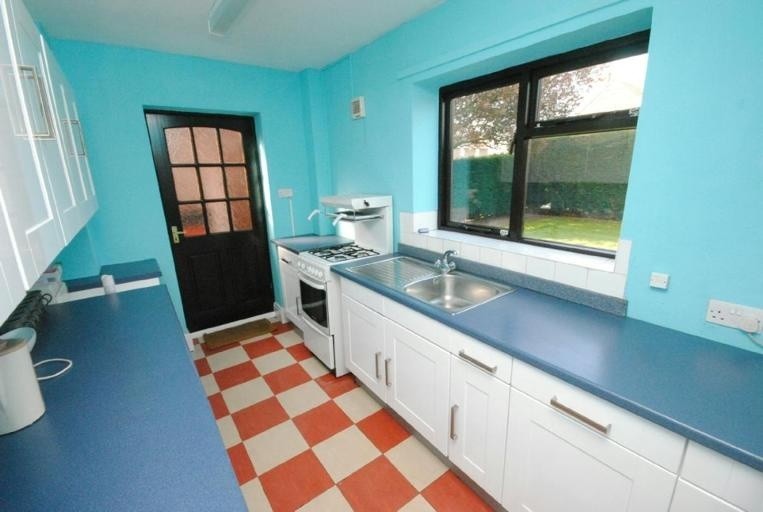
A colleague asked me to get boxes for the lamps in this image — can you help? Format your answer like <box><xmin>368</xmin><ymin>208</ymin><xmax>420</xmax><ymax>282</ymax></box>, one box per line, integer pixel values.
<box><xmin>207</xmin><ymin>2</ymin><xmax>249</xmax><ymax>37</ymax></box>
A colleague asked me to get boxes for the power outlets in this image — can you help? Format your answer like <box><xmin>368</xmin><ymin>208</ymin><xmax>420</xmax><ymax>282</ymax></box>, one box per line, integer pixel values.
<box><xmin>706</xmin><ymin>299</ymin><xmax>763</xmax><ymax>334</ymax></box>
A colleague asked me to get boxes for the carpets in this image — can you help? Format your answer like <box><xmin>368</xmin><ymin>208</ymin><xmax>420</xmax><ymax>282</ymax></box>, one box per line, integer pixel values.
<box><xmin>204</xmin><ymin>318</ymin><xmax>277</xmax><ymax>350</ymax></box>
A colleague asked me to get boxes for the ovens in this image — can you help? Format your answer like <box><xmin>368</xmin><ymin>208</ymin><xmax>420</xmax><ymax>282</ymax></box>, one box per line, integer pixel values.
<box><xmin>296</xmin><ymin>259</ymin><xmax>332</xmax><ymax>335</ymax></box>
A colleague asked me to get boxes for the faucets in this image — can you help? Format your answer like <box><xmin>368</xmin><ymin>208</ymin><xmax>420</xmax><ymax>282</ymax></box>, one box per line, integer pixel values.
<box><xmin>435</xmin><ymin>251</ymin><xmax>457</xmax><ymax>274</ymax></box>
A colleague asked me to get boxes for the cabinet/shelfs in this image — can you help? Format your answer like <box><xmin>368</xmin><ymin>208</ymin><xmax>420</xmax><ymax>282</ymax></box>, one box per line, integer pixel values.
<box><xmin>447</xmin><ymin>325</ymin><xmax>514</xmax><ymax>512</ymax></box>
<box><xmin>668</xmin><ymin>439</ymin><xmax>763</xmax><ymax>512</ymax></box>
<box><xmin>1</xmin><ymin>0</ymin><xmax>99</xmax><ymax>329</ymax></box>
<box><xmin>500</xmin><ymin>357</ymin><xmax>688</xmax><ymax>512</ymax></box>
<box><xmin>339</xmin><ymin>277</ymin><xmax>447</xmax><ymax>468</ymax></box>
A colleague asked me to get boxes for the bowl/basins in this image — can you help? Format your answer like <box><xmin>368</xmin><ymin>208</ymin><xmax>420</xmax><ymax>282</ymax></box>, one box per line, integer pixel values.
<box><xmin>0</xmin><ymin>327</ymin><xmax>37</xmax><ymax>354</ymax></box>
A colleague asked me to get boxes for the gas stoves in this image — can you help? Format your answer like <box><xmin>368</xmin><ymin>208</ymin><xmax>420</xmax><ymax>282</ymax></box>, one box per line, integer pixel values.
<box><xmin>299</xmin><ymin>242</ymin><xmax>387</xmax><ymax>267</ymax></box>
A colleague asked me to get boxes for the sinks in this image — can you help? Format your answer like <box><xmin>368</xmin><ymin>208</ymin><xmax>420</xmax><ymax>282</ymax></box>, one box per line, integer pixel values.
<box><xmin>403</xmin><ymin>275</ymin><xmax>517</xmax><ymax>316</ymax></box>
